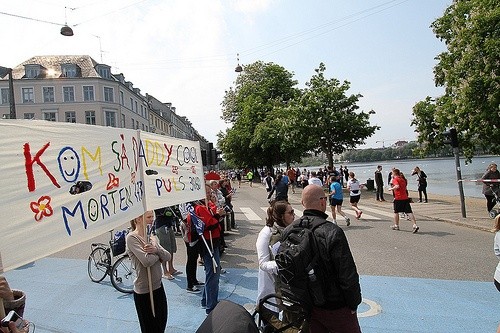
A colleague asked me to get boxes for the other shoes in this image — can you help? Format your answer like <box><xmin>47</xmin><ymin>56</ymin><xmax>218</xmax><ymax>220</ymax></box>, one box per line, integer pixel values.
<box><xmin>201</xmin><ymin>304</ymin><xmax>213</xmax><ymax>315</ymax></box>
<box><xmin>390</xmin><ymin>225</ymin><xmax>399</xmax><ymax>230</ymax></box>
<box><xmin>220</xmin><ymin>269</ymin><xmax>226</xmax><ymax>274</ymax></box>
<box><xmin>345</xmin><ymin>218</ymin><xmax>350</xmax><ymax>226</ymax></box>
<box><xmin>411</xmin><ymin>225</ymin><xmax>419</xmax><ymax>234</ymax></box>
<box><xmin>356</xmin><ymin>211</ymin><xmax>362</xmax><ymax>218</ymax></box>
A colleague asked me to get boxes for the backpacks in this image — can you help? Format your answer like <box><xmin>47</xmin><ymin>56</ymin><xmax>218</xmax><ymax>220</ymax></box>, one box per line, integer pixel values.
<box><xmin>274</xmin><ymin>216</ymin><xmax>332</xmax><ymax>312</ymax></box>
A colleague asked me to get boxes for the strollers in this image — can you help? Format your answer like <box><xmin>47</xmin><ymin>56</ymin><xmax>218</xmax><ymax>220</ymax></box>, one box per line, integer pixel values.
<box><xmin>488</xmin><ymin>184</ymin><xmax>500</xmax><ymax>219</ymax></box>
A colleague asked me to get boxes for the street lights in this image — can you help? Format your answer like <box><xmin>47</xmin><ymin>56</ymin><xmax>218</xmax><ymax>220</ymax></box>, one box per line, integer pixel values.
<box><xmin>0</xmin><ymin>65</ymin><xmax>17</xmax><ymax>120</ymax></box>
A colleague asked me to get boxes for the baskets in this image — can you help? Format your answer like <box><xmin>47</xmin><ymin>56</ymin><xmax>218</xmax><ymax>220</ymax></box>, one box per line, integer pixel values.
<box><xmin>110</xmin><ymin>239</ymin><xmax>126</xmax><ymax>257</ymax></box>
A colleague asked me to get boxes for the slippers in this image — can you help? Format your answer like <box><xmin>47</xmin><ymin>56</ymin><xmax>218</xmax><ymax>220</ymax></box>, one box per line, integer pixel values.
<box><xmin>163</xmin><ymin>274</ymin><xmax>175</xmax><ymax>280</ymax></box>
<box><xmin>172</xmin><ymin>270</ymin><xmax>183</xmax><ymax>274</ymax></box>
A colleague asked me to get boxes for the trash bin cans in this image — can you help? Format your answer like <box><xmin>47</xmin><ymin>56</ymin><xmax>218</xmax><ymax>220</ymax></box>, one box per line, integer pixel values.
<box><xmin>367</xmin><ymin>180</ymin><xmax>374</xmax><ymax>191</ymax></box>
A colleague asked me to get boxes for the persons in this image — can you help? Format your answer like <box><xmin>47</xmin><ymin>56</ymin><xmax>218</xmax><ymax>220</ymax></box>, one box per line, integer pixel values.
<box><xmin>126</xmin><ymin>164</ymin><xmax>411</xmax><ymax>333</ymax></box>
<box><xmin>493</xmin><ymin>214</ymin><xmax>500</xmax><ymax>293</ymax></box>
<box><xmin>0</xmin><ymin>277</ymin><xmax>32</xmax><ymax>333</ymax></box>
<box><xmin>411</xmin><ymin>166</ymin><xmax>428</xmax><ymax>203</ymax></box>
<box><xmin>374</xmin><ymin>165</ymin><xmax>386</xmax><ymax>202</ymax></box>
<box><xmin>276</xmin><ymin>185</ymin><xmax>362</xmax><ymax>333</ymax></box>
<box><xmin>328</xmin><ymin>176</ymin><xmax>350</xmax><ymax>226</ymax></box>
<box><xmin>481</xmin><ymin>162</ymin><xmax>500</xmax><ymax>220</ymax></box>
<box><xmin>389</xmin><ymin>169</ymin><xmax>419</xmax><ymax>234</ymax></box>
<box><xmin>256</xmin><ymin>200</ymin><xmax>296</xmax><ymax>333</ymax></box>
<box><xmin>346</xmin><ymin>172</ymin><xmax>362</xmax><ymax>220</ymax></box>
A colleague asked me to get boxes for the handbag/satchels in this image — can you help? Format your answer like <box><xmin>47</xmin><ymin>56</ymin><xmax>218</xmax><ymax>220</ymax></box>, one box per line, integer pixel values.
<box><xmin>352</xmin><ymin>190</ymin><xmax>359</xmax><ymax>194</ymax></box>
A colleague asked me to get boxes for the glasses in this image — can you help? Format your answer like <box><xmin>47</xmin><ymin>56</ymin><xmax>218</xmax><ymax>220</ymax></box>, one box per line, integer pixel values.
<box><xmin>283</xmin><ymin>209</ymin><xmax>294</xmax><ymax>216</ymax></box>
<box><xmin>320</xmin><ymin>197</ymin><xmax>327</xmax><ymax>201</ymax></box>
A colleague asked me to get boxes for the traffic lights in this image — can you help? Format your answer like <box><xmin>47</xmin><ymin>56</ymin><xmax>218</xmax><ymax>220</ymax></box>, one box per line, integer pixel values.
<box><xmin>441</xmin><ymin>128</ymin><xmax>455</xmax><ymax>148</ymax></box>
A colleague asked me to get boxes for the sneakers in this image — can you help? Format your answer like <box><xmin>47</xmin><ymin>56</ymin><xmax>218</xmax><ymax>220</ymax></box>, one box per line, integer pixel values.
<box><xmin>186</xmin><ymin>285</ymin><xmax>202</xmax><ymax>294</ymax></box>
<box><xmin>195</xmin><ymin>281</ymin><xmax>206</xmax><ymax>286</ymax></box>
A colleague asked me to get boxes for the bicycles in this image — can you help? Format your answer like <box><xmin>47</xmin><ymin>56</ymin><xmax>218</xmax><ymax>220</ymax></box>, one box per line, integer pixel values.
<box><xmin>86</xmin><ymin>230</ymin><xmax>137</xmax><ymax>294</ymax></box>
